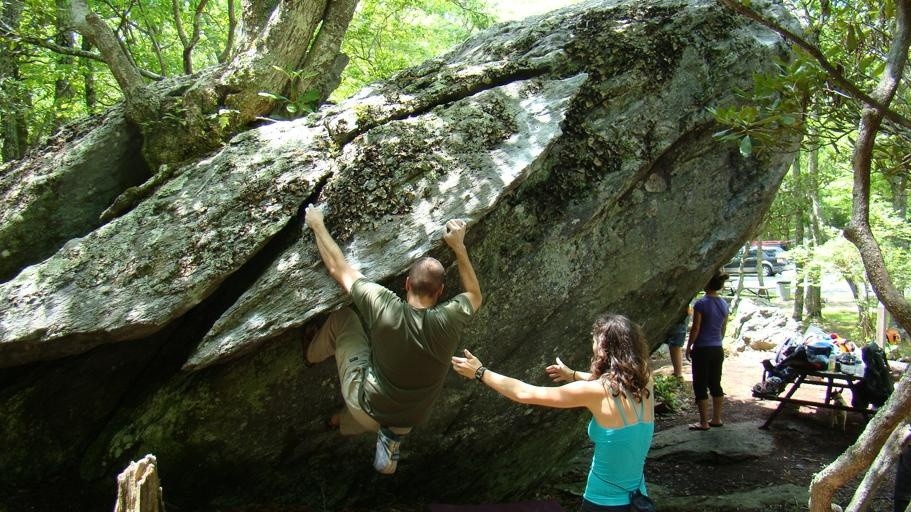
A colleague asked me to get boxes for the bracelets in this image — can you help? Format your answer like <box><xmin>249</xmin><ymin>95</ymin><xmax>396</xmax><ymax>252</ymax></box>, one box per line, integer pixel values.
<box><xmin>572</xmin><ymin>371</ymin><xmax>576</xmax><ymax>382</ymax></box>
<box><xmin>475</xmin><ymin>365</ymin><xmax>487</xmax><ymax>383</ymax></box>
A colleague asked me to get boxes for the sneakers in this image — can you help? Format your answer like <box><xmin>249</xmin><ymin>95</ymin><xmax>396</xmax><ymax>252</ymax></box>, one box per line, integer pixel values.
<box><xmin>303</xmin><ymin>323</ymin><xmax>318</xmax><ymax>368</ymax></box>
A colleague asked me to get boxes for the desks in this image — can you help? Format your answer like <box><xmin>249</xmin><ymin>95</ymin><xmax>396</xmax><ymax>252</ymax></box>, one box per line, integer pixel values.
<box><xmin>759</xmin><ymin>364</ymin><xmax>877</xmax><ymax>430</ymax></box>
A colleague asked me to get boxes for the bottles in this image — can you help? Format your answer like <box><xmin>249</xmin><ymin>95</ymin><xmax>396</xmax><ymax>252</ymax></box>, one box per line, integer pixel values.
<box><xmin>855</xmin><ymin>360</ymin><xmax>862</xmax><ymax>377</ymax></box>
<box><xmin>826</xmin><ymin>351</ymin><xmax>836</xmax><ymax>372</ymax></box>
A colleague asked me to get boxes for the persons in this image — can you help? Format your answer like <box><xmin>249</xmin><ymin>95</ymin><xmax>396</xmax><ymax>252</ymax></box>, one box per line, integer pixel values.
<box><xmin>302</xmin><ymin>202</ymin><xmax>483</xmax><ymax>476</ymax></box>
<box><xmin>684</xmin><ymin>270</ymin><xmax>730</xmax><ymax>430</ymax></box>
<box><xmin>665</xmin><ymin>303</ymin><xmax>694</xmax><ymax>379</ymax></box>
<box><xmin>450</xmin><ymin>313</ymin><xmax>658</xmax><ymax>512</ymax></box>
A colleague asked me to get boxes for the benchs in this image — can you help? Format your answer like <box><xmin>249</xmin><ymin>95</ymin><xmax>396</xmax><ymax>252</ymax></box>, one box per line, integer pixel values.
<box><xmin>717</xmin><ymin>279</ymin><xmax>779</xmax><ymax>307</ymax></box>
<box><xmin>752</xmin><ymin>382</ymin><xmax>789</xmax><ymax>401</ymax></box>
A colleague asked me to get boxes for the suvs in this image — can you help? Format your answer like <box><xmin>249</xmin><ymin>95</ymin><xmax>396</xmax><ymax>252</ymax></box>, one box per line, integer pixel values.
<box><xmin>722</xmin><ymin>246</ymin><xmax>794</xmax><ymax>275</ymax></box>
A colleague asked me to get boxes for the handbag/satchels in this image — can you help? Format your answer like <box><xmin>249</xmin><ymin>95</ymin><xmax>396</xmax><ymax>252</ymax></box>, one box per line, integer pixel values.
<box><xmin>373</xmin><ymin>424</ymin><xmax>401</xmax><ymax>474</ymax></box>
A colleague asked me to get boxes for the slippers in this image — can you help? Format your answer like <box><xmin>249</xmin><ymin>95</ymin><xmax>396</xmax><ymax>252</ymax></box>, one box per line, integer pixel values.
<box><xmin>688</xmin><ymin>420</ymin><xmax>722</xmax><ymax>431</ymax></box>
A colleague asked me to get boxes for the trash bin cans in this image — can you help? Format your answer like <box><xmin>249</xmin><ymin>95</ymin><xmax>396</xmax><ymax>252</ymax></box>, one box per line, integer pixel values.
<box><xmin>776</xmin><ymin>281</ymin><xmax>791</xmax><ymax>301</ymax></box>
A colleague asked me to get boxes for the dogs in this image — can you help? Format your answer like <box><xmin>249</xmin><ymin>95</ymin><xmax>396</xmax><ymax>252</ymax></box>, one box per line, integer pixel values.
<box><xmin>829</xmin><ymin>386</ymin><xmax>848</xmax><ymax>432</ymax></box>
